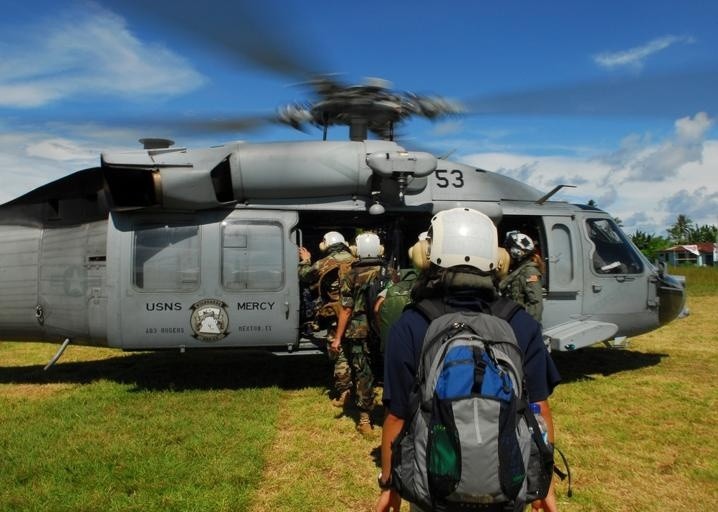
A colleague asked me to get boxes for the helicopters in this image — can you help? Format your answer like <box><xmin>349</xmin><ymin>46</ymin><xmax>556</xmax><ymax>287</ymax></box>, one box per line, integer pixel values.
<box><xmin>0</xmin><ymin>0</ymin><xmax>690</xmax><ymax>376</ymax></box>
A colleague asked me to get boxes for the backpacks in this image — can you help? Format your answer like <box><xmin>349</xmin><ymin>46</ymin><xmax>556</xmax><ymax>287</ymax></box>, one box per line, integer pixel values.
<box><xmin>364</xmin><ymin>268</ymin><xmax>391</xmax><ymax>335</ymax></box>
<box><xmin>318</xmin><ymin>258</ymin><xmax>347</xmax><ymax>318</ymax></box>
<box><xmin>380</xmin><ymin>271</ymin><xmax>422</xmax><ymax>352</ymax></box>
<box><xmin>393</xmin><ymin>298</ymin><xmax>554</xmax><ymax>511</ymax></box>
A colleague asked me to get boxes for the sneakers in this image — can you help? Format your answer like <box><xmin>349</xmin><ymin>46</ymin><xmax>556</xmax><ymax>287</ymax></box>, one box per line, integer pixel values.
<box><xmin>333</xmin><ymin>388</ymin><xmax>375</xmax><ymax>442</ymax></box>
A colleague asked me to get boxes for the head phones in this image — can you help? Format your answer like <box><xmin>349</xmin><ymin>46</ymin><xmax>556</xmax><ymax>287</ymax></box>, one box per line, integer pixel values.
<box><xmin>510</xmin><ymin>247</ymin><xmax>524</xmax><ymax>260</ymax></box>
<box><xmin>319</xmin><ymin>240</ymin><xmax>349</xmax><ymax>253</ymax></box>
<box><xmin>407</xmin><ymin>225</ymin><xmax>511</xmax><ymax>277</ymax></box>
<box><xmin>349</xmin><ymin>244</ymin><xmax>385</xmax><ymax>258</ymax></box>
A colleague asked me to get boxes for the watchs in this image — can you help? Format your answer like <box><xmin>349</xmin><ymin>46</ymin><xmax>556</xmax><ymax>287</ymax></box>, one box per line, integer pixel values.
<box><xmin>376</xmin><ymin>473</ymin><xmax>390</xmax><ymax>491</ymax></box>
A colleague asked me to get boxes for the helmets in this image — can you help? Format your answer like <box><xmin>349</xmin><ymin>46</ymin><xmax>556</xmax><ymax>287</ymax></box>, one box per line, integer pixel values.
<box><xmin>323</xmin><ymin>231</ymin><xmax>346</xmax><ymax>247</ymax></box>
<box><xmin>426</xmin><ymin>207</ymin><xmax>501</xmax><ymax>273</ymax></box>
<box><xmin>355</xmin><ymin>232</ymin><xmax>381</xmax><ymax>259</ymax></box>
<box><xmin>507</xmin><ymin>232</ymin><xmax>535</xmax><ymax>264</ymax></box>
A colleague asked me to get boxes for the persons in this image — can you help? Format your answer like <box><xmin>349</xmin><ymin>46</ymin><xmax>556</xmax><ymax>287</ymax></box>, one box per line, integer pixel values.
<box><xmin>372</xmin><ymin>238</ymin><xmax>429</xmax><ymax>359</ymax></box>
<box><xmin>375</xmin><ymin>206</ymin><xmax>566</xmax><ymax>512</ymax></box>
<box><xmin>297</xmin><ymin>229</ymin><xmax>355</xmax><ymax>409</ymax></box>
<box><xmin>299</xmin><ymin>283</ymin><xmax>313</xmax><ymax>340</ymax></box>
<box><xmin>329</xmin><ymin>232</ymin><xmax>395</xmax><ymax>439</ymax></box>
<box><xmin>498</xmin><ymin>230</ymin><xmax>546</xmax><ymax>324</ymax></box>
<box><xmin>375</xmin><ymin>268</ymin><xmax>415</xmax><ymax>313</ymax></box>
